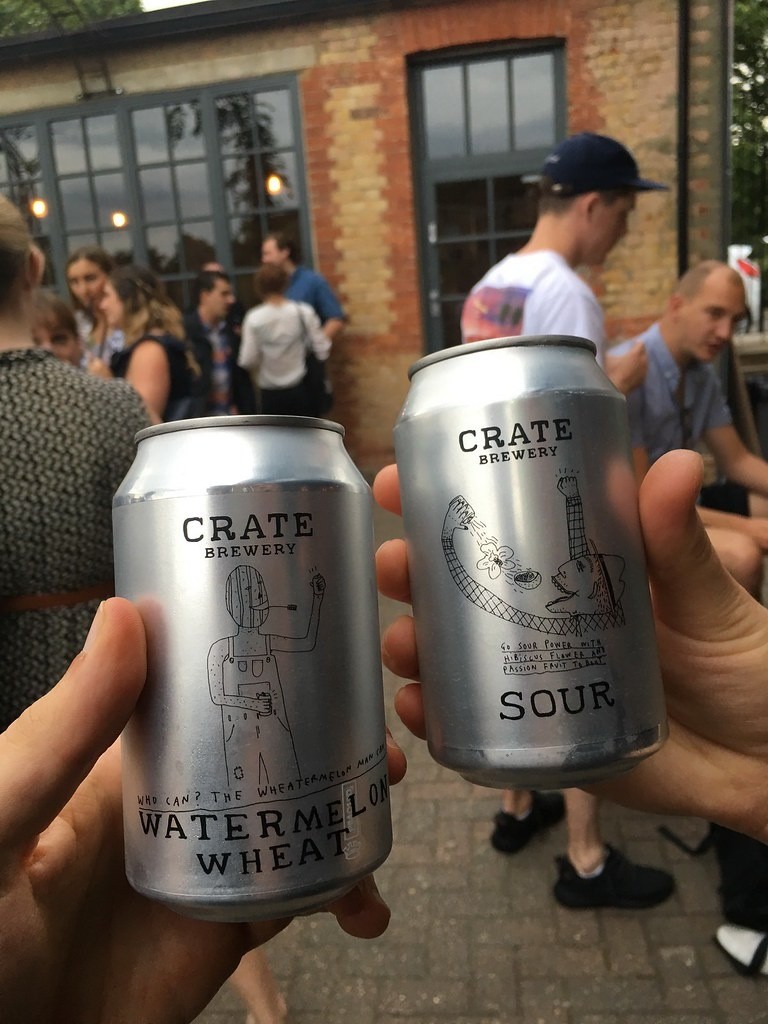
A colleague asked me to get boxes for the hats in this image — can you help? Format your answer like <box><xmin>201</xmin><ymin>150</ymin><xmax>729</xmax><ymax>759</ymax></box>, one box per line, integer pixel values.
<box><xmin>537</xmin><ymin>130</ymin><xmax>670</xmax><ymax>193</ymax></box>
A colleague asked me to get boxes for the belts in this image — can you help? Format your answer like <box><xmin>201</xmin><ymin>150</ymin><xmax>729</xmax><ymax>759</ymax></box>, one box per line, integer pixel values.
<box><xmin>0</xmin><ymin>581</ymin><xmax>115</xmax><ymax>612</ymax></box>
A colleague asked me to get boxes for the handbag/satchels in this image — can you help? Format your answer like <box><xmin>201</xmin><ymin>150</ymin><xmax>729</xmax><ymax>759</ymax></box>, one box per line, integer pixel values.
<box><xmin>302</xmin><ymin>341</ymin><xmax>335</xmax><ymax>418</ymax></box>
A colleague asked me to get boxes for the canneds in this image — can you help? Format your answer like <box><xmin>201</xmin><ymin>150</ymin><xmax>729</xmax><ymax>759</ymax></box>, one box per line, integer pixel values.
<box><xmin>390</xmin><ymin>334</ymin><xmax>668</xmax><ymax>792</ymax></box>
<box><xmin>111</xmin><ymin>414</ymin><xmax>392</xmax><ymax>924</ymax></box>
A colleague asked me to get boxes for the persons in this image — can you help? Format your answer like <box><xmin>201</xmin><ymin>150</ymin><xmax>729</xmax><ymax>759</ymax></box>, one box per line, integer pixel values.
<box><xmin>31</xmin><ymin>234</ymin><xmax>349</xmax><ymax>427</ymax></box>
<box><xmin>0</xmin><ymin>195</ymin><xmax>152</xmax><ymax>733</ymax></box>
<box><xmin>458</xmin><ymin>133</ymin><xmax>675</xmax><ymax>911</ymax></box>
<box><xmin>604</xmin><ymin>261</ymin><xmax>766</xmax><ymax>607</ymax></box>
<box><xmin>0</xmin><ymin>448</ymin><xmax>767</xmax><ymax>1024</ymax></box>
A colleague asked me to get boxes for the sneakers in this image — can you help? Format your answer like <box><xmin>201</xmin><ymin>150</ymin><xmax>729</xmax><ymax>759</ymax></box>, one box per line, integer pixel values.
<box><xmin>552</xmin><ymin>842</ymin><xmax>677</xmax><ymax>912</ymax></box>
<box><xmin>489</xmin><ymin>790</ymin><xmax>568</xmax><ymax>858</ymax></box>
<box><xmin>712</xmin><ymin>921</ymin><xmax>767</xmax><ymax>980</ymax></box>
